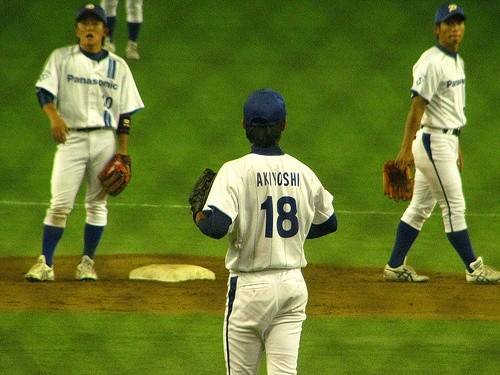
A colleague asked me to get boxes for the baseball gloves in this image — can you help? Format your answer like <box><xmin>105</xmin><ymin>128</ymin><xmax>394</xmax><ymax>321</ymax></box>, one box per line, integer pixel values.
<box><xmin>188</xmin><ymin>168</ymin><xmax>218</xmax><ymax>213</ymax></box>
<box><xmin>382</xmin><ymin>157</ymin><xmax>414</xmax><ymax>202</ymax></box>
<box><xmin>97</xmin><ymin>153</ymin><xmax>132</xmax><ymax>197</ymax></box>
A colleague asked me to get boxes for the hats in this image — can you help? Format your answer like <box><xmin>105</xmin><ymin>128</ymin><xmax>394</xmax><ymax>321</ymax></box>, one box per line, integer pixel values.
<box><xmin>76</xmin><ymin>4</ymin><xmax>106</xmax><ymax>23</ymax></box>
<box><xmin>243</xmin><ymin>89</ymin><xmax>286</xmax><ymax>128</ymax></box>
<box><xmin>434</xmin><ymin>4</ymin><xmax>466</xmax><ymax>24</ymax></box>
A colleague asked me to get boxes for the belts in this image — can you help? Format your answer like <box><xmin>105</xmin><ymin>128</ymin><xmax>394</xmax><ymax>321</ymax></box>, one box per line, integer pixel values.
<box><xmin>66</xmin><ymin>125</ymin><xmax>114</xmax><ymax>135</ymax></box>
<box><xmin>420</xmin><ymin>126</ymin><xmax>462</xmax><ymax>136</ymax></box>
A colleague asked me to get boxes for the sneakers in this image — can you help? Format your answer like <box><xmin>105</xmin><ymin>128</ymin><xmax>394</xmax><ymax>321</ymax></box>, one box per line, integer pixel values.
<box><xmin>124</xmin><ymin>39</ymin><xmax>140</xmax><ymax>60</ymax></box>
<box><xmin>383</xmin><ymin>255</ymin><xmax>429</xmax><ymax>282</ymax></box>
<box><xmin>25</xmin><ymin>254</ymin><xmax>55</xmax><ymax>281</ymax></box>
<box><xmin>466</xmin><ymin>256</ymin><xmax>500</xmax><ymax>284</ymax></box>
<box><xmin>76</xmin><ymin>255</ymin><xmax>99</xmax><ymax>283</ymax></box>
<box><xmin>103</xmin><ymin>37</ymin><xmax>115</xmax><ymax>50</ymax></box>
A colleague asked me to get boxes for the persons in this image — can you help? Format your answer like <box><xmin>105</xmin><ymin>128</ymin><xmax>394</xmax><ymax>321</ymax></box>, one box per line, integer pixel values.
<box><xmin>100</xmin><ymin>0</ymin><xmax>144</xmax><ymax>59</ymax></box>
<box><xmin>188</xmin><ymin>89</ymin><xmax>338</xmax><ymax>375</ymax></box>
<box><xmin>24</xmin><ymin>3</ymin><xmax>146</xmax><ymax>282</ymax></box>
<box><xmin>383</xmin><ymin>2</ymin><xmax>500</xmax><ymax>283</ymax></box>
